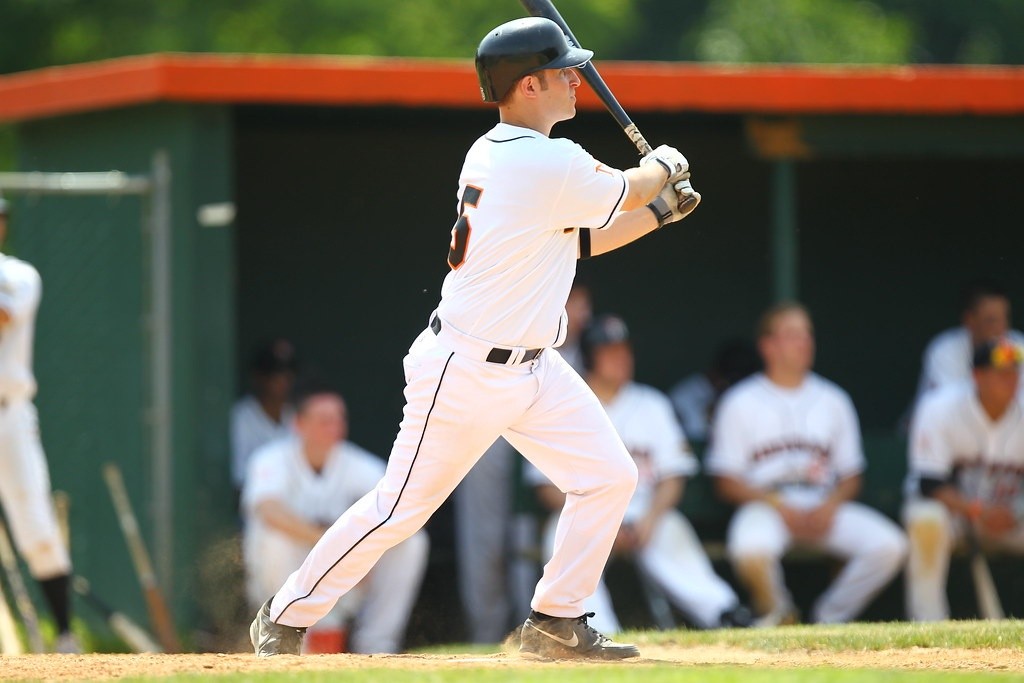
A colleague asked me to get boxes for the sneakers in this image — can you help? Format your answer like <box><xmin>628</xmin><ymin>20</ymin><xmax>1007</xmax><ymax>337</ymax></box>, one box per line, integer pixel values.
<box><xmin>249</xmin><ymin>595</ymin><xmax>306</xmax><ymax>659</ymax></box>
<box><xmin>517</xmin><ymin>612</ymin><xmax>640</xmax><ymax>663</ymax></box>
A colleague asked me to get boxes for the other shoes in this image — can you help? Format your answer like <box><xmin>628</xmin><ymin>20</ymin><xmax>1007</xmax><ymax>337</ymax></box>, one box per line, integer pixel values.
<box><xmin>723</xmin><ymin>606</ymin><xmax>755</xmax><ymax>629</ymax></box>
<box><xmin>55</xmin><ymin>632</ymin><xmax>81</xmax><ymax>654</ymax></box>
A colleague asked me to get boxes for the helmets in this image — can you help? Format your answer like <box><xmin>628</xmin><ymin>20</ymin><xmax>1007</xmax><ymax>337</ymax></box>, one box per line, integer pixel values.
<box><xmin>579</xmin><ymin>312</ymin><xmax>631</xmax><ymax>351</ymax></box>
<box><xmin>475</xmin><ymin>17</ymin><xmax>594</xmax><ymax>103</ymax></box>
<box><xmin>973</xmin><ymin>338</ymin><xmax>1023</xmax><ymax>369</ymax></box>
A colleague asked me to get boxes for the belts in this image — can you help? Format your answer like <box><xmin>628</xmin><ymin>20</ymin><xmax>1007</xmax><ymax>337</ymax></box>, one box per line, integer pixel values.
<box><xmin>430</xmin><ymin>315</ymin><xmax>546</xmax><ymax>365</ymax></box>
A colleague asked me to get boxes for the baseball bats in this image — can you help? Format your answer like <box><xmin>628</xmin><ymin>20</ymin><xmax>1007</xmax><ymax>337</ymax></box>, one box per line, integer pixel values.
<box><xmin>517</xmin><ymin>0</ymin><xmax>702</xmax><ymax>215</ymax></box>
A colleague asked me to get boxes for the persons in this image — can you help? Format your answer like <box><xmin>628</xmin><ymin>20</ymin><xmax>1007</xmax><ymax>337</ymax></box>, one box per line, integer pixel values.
<box><xmin>1</xmin><ymin>193</ymin><xmax>72</xmax><ymax>655</ymax></box>
<box><xmin>249</xmin><ymin>17</ymin><xmax>702</xmax><ymax>655</ymax></box>
<box><xmin>228</xmin><ymin>294</ymin><xmax>1024</xmax><ymax>652</ymax></box>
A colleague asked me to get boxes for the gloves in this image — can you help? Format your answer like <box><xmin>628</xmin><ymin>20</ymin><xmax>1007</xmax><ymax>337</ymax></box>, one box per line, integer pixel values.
<box><xmin>639</xmin><ymin>143</ymin><xmax>689</xmax><ymax>182</ymax></box>
<box><xmin>645</xmin><ymin>172</ymin><xmax>702</xmax><ymax>229</ymax></box>
